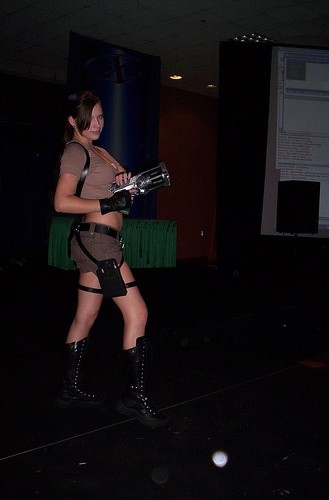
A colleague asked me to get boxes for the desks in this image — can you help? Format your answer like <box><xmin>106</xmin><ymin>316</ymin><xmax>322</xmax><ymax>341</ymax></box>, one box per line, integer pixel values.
<box><xmin>44</xmin><ymin>216</ymin><xmax>178</xmax><ymax>282</ymax></box>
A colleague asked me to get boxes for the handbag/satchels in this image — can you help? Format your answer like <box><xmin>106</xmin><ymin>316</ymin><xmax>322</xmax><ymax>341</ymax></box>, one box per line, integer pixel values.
<box><xmin>95</xmin><ymin>259</ymin><xmax>128</xmax><ymax>299</ymax></box>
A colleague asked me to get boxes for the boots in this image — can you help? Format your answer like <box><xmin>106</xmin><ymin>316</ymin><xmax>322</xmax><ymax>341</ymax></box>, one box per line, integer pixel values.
<box><xmin>115</xmin><ymin>335</ymin><xmax>168</xmax><ymax>428</ymax></box>
<box><xmin>53</xmin><ymin>336</ymin><xmax>105</xmax><ymax>407</ymax></box>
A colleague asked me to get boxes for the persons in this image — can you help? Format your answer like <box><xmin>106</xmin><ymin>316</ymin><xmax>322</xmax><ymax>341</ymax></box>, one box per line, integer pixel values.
<box><xmin>53</xmin><ymin>92</ymin><xmax>171</xmax><ymax>426</ymax></box>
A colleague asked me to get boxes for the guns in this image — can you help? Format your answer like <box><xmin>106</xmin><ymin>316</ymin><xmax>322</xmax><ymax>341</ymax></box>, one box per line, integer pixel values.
<box><xmin>106</xmin><ymin>162</ymin><xmax>171</xmax><ymax>214</ymax></box>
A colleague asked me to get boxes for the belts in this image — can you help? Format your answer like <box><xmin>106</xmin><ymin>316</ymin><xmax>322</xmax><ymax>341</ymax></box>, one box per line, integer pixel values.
<box><xmin>76</xmin><ymin>223</ymin><xmax>123</xmax><ymax>242</ymax></box>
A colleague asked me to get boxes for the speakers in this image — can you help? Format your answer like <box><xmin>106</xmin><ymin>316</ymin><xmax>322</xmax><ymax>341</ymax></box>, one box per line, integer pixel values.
<box><xmin>276</xmin><ymin>180</ymin><xmax>320</xmax><ymax>235</ymax></box>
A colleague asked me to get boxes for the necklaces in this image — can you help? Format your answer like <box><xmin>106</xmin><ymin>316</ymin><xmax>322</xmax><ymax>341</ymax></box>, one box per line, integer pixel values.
<box><xmin>88</xmin><ymin>145</ymin><xmax>118</xmax><ymax>170</ymax></box>
